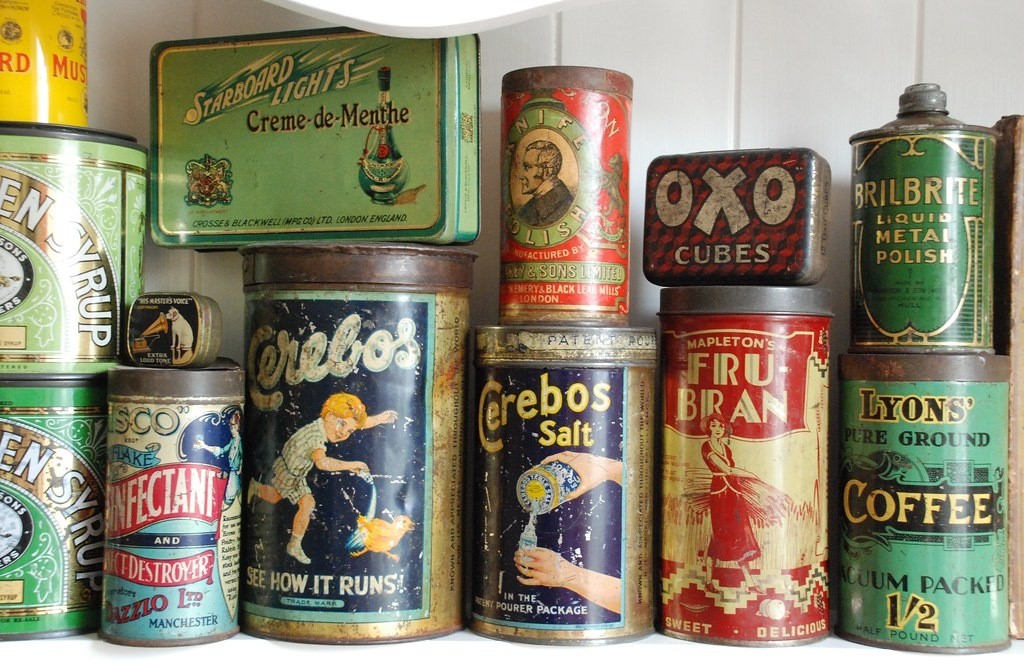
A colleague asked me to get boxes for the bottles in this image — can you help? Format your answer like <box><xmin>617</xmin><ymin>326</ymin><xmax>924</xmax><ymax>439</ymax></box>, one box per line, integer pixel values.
<box><xmin>0</xmin><ymin>1</ymin><xmax>1024</xmax><ymax>657</ymax></box>
